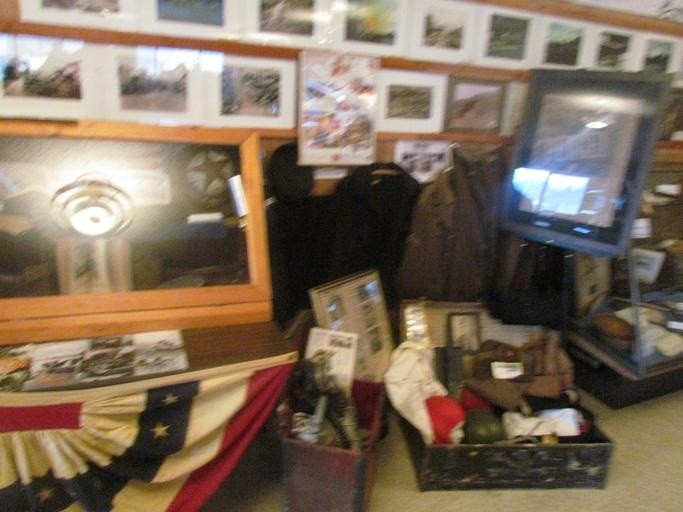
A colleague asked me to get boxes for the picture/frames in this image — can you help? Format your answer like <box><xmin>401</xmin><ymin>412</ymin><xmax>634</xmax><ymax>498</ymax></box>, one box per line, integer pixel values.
<box><xmin>306</xmin><ymin>267</ymin><xmax>394</xmax><ymax>382</ymax></box>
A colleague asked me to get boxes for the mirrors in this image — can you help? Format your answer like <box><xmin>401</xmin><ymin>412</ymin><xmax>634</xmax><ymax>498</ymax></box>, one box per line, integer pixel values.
<box><xmin>1</xmin><ymin>135</ymin><xmax>255</xmax><ymax>303</ymax></box>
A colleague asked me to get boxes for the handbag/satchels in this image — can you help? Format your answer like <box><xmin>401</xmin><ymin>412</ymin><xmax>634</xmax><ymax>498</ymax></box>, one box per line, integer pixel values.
<box><xmin>263</xmin><ymin>158</ymin><xmax>423</xmax><ymax>325</ymax></box>
<box><xmin>394</xmin><ymin>144</ymin><xmax>534</xmax><ymax>316</ymax></box>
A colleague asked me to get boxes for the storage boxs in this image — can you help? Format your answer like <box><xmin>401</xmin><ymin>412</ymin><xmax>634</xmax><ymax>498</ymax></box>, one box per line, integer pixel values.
<box><xmin>279</xmin><ymin>377</ymin><xmax>387</xmax><ymax>512</ymax></box>
<box><xmin>409</xmin><ymin>422</ymin><xmax>616</xmax><ymax>492</ymax></box>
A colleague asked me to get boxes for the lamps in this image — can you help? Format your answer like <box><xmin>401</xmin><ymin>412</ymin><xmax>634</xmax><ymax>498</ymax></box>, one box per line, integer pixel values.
<box><xmin>44</xmin><ymin>172</ymin><xmax>143</xmax><ymax>245</ymax></box>
